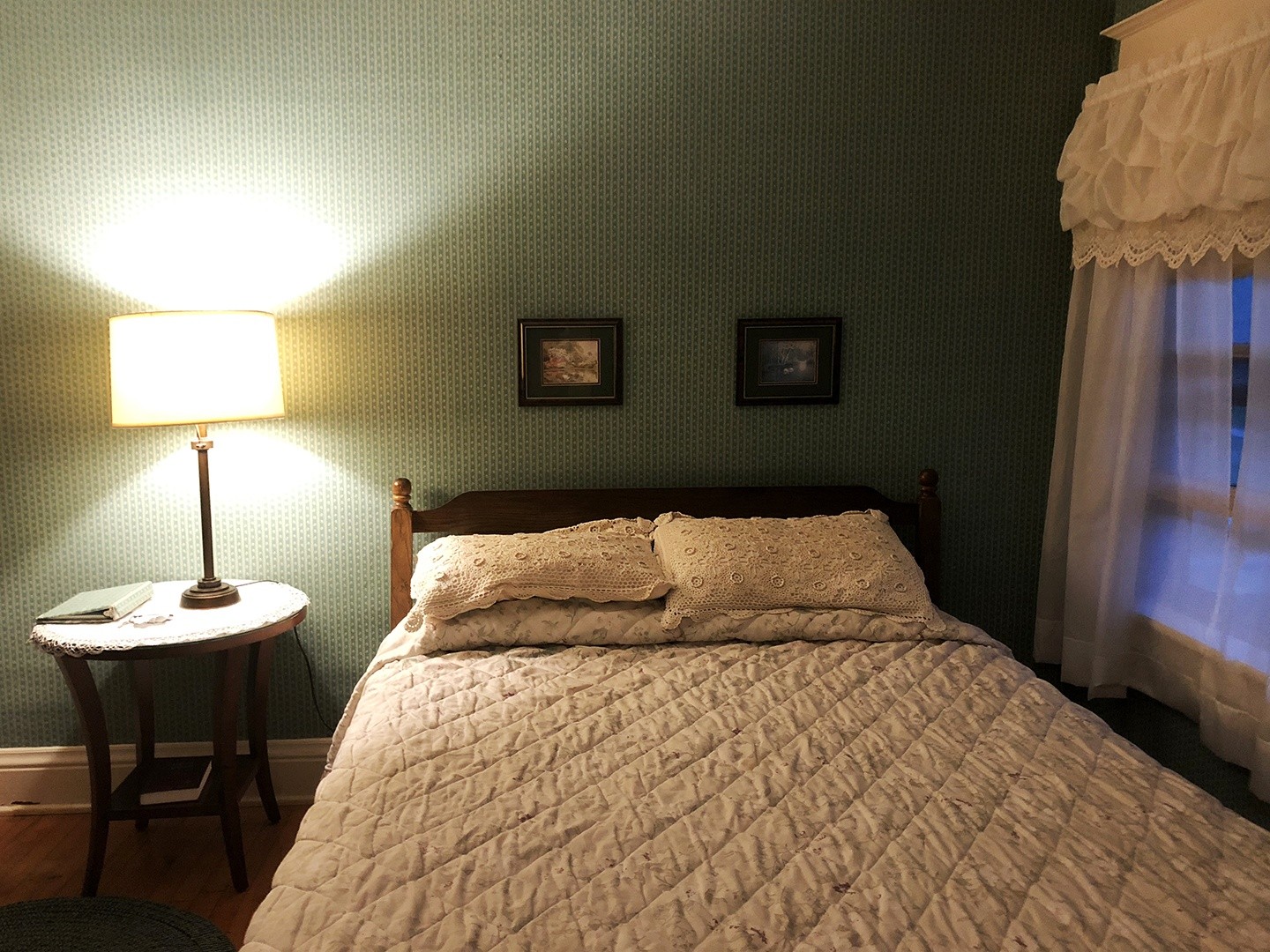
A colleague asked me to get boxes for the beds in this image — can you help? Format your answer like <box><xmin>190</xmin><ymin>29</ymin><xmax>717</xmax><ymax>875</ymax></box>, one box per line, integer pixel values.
<box><xmin>237</xmin><ymin>476</ymin><xmax>1270</xmax><ymax>952</ymax></box>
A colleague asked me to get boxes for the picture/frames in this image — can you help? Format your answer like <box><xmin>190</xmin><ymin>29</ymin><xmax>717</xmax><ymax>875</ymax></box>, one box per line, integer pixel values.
<box><xmin>735</xmin><ymin>316</ymin><xmax>843</xmax><ymax>407</ymax></box>
<box><xmin>517</xmin><ymin>318</ymin><xmax>624</xmax><ymax>408</ymax></box>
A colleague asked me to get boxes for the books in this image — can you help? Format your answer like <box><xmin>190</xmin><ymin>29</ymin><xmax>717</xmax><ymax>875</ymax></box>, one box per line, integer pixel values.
<box><xmin>36</xmin><ymin>581</ymin><xmax>153</xmax><ymax>624</ymax></box>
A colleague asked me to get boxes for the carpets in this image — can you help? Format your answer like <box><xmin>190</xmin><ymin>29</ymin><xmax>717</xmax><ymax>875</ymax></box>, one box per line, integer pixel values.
<box><xmin>0</xmin><ymin>898</ymin><xmax>239</xmax><ymax>952</ymax></box>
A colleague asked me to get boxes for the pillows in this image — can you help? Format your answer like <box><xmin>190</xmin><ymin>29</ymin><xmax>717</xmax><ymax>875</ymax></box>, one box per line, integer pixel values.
<box><xmin>409</xmin><ymin>516</ymin><xmax>672</xmax><ymax>620</ymax></box>
<box><xmin>653</xmin><ymin>508</ymin><xmax>947</xmax><ymax>631</ymax></box>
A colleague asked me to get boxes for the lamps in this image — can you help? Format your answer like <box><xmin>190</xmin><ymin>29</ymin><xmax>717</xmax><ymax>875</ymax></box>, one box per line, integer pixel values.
<box><xmin>107</xmin><ymin>310</ymin><xmax>287</xmax><ymax>609</ymax></box>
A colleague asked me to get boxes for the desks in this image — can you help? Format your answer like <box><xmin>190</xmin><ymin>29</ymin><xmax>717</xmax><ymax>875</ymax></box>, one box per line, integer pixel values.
<box><xmin>30</xmin><ymin>581</ymin><xmax>309</xmax><ymax>900</ymax></box>
